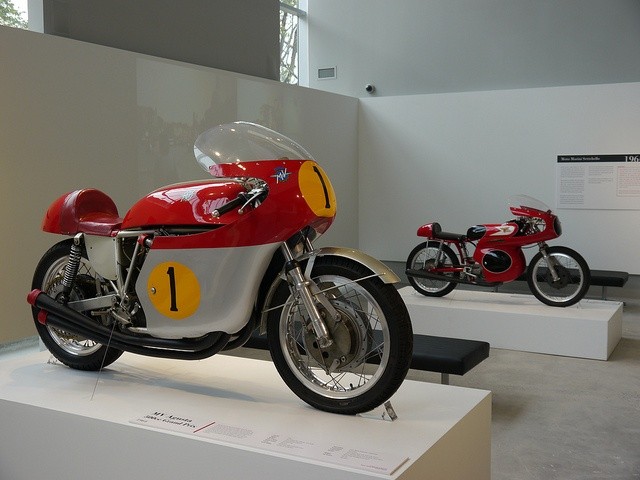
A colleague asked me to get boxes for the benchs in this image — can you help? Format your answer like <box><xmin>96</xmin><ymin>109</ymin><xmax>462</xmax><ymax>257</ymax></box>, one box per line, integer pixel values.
<box><xmin>514</xmin><ymin>265</ymin><xmax>629</xmax><ymax>301</ymax></box>
<box><xmin>242</xmin><ymin>318</ymin><xmax>490</xmax><ymax>385</ymax></box>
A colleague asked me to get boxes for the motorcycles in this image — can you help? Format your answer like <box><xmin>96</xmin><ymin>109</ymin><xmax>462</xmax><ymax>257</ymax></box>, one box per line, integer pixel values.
<box><xmin>405</xmin><ymin>195</ymin><xmax>590</xmax><ymax>308</ymax></box>
<box><xmin>25</xmin><ymin>119</ymin><xmax>413</xmax><ymax>415</ymax></box>
